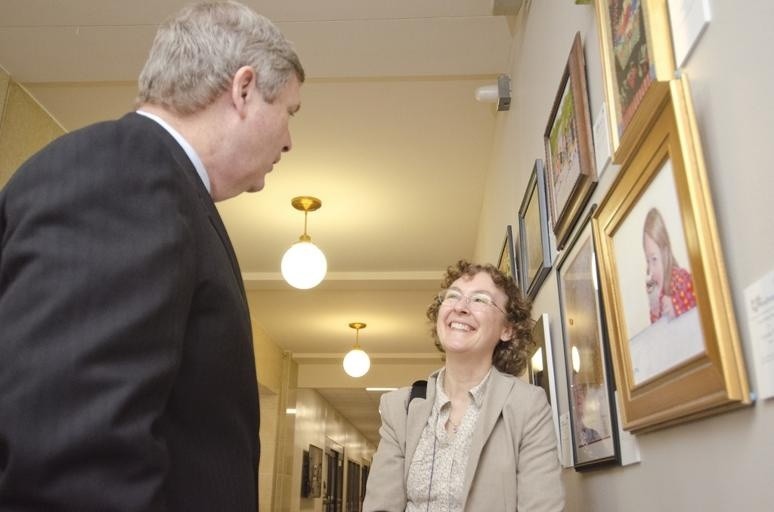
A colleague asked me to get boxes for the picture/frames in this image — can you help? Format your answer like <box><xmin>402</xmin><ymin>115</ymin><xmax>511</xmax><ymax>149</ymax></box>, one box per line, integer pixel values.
<box><xmin>496</xmin><ymin>225</ymin><xmax>519</xmax><ymax>282</ymax></box>
<box><xmin>595</xmin><ymin>0</ymin><xmax>676</xmax><ymax>163</ymax></box>
<box><xmin>590</xmin><ymin>76</ymin><xmax>751</xmax><ymax>438</ymax></box>
<box><xmin>305</xmin><ymin>444</ymin><xmax>323</xmax><ymax>499</ymax></box>
<box><xmin>527</xmin><ymin>312</ymin><xmax>561</xmax><ymax>460</ymax></box>
<box><xmin>543</xmin><ymin>32</ymin><xmax>599</xmax><ymax>249</ymax></box>
<box><xmin>517</xmin><ymin>160</ymin><xmax>552</xmax><ymax>302</ymax></box>
<box><xmin>556</xmin><ymin>203</ymin><xmax>642</xmax><ymax>470</ymax></box>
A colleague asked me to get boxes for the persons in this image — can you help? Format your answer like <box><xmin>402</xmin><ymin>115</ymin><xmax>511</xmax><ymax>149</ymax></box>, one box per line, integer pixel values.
<box><xmin>359</xmin><ymin>258</ymin><xmax>567</xmax><ymax>511</ymax></box>
<box><xmin>641</xmin><ymin>207</ymin><xmax>698</xmax><ymax>325</ymax></box>
<box><xmin>551</xmin><ymin>108</ymin><xmax>579</xmax><ymax>187</ymax></box>
<box><xmin>637</xmin><ymin>59</ymin><xmax>649</xmax><ymax>78</ymax></box>
<box><xmin>572</xmin><ymin>368</ymin><xmax>603</xmax><ymax>448</ymax></box>
<box><xmin>1</xmin><ymin>2</ymin><xmax>307</xmax><ymax>510</ymax></box>
<box><xmin>621</xmin><ymin>58</ymin><xmax>643</xmax><ymax>112</ymax></box>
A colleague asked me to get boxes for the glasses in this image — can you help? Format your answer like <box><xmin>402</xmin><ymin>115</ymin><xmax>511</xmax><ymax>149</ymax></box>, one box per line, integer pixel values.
<box><xmin>437</xmin><ymin>288</ymin><xmax>508</xmax><ymax>319</ymax></box>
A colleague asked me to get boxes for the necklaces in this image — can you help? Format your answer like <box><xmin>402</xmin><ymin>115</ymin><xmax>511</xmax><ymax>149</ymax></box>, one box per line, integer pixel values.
<box><xmin>447</xmin><ymin>418</ymin><xmax>458</xmax><ymax>433</ymax></box>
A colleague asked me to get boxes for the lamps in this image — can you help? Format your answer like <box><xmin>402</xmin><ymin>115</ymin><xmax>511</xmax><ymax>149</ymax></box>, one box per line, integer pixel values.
<box><xmin>280</xmin><ymin>196</ymin><xmax>327</xmax><ymax>291</ymax></box>
<box><xmin>474</xmin><ymin>72</ymin><xmax>511</xmax><ymax>112</ymax></box>
<box><xmin>343</xmin><ymin>323</ymin><xmax>371</xmax><ymax>377</ymax></box>
